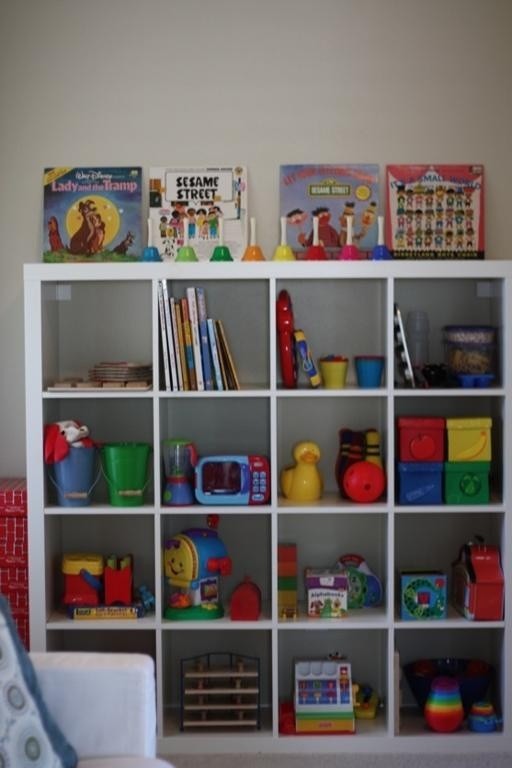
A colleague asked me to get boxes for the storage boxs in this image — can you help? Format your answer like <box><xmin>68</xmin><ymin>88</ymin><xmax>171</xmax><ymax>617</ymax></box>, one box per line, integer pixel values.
<box><xmin>397</xmin><ymin>415</ymin><xmax>493</xmax><ymax>506</ymax></box>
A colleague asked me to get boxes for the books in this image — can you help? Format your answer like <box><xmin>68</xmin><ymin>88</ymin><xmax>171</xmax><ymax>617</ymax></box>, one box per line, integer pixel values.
<box><xmin>147</xmin><ymin>168</ymin><xmax>249</xmax><ymax>262</ymax></box>
<box><xmin>280</xmin><ymin>165</ymin><xmax>386</xmax><ymax>259</ymax></box>
<box><xmin>385</xmin><ymin>163</ymin><xmax>487</xmax><ymax>260</ymax></box>
<box><xmin>43</xmin><ymin>167</ymin><xmax>145</xmax><ymax>263</ymax></box>
<box><xmin>88</xmin><ymin>361</ymin><xmax>150</xmax><ymax>381</ymax></box>
<box><xmin>157</xmin><ymin>280</ymin><xmax>240</xmax><ymax>389</ymax></box>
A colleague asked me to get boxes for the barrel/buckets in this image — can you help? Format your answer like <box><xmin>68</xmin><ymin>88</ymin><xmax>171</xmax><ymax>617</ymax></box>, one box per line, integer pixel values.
<box><xmin>96</xmin><ymin>442</ymin><xmax>154</xmax><ymax>506</ymax></box>
<box><xmin>46</xmin><ymin>444</ymin><xmax>102</xmax><ymax>506</ymax></box>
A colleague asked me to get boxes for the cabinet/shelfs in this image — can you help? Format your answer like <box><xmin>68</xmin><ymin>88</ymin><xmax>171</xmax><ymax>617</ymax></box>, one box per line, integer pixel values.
<box><xmin>20</xmin><ymin>260</ymin><xmax>511</xmax><ymax>757</ymax></box>
